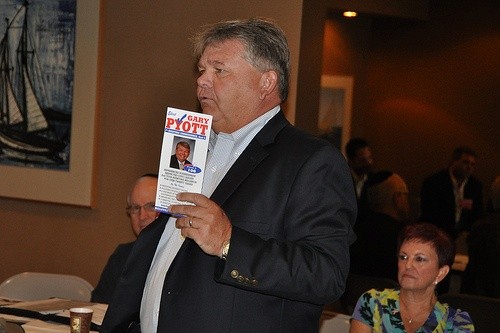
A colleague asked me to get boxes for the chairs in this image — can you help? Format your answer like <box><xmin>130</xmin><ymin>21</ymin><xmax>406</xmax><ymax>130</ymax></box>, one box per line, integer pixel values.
<box><xmin>318</xmin><ymin>312</ymin><xmax>351</xmax><ymax>333</ymax></box>
<box><xmin>436</xmin><ymin>293</ymin><xmax>500</xmax><ymax>333</ymax></box>
<box><xmin>328</xmin><ymin>273</ymin><xmax>402</xmax><ymax>318</ymax></box>
<box><xmin>0</xmin><ymin>272</ymin><xmax>94</xmax><ymax>301</ymax></box>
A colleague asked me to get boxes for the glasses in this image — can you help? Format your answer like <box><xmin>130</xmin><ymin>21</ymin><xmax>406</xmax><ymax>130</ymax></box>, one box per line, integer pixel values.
<box><xmin>126</xmin><ymin>202</ymin><xmax>155</xmax><ymax>214</ymax></box>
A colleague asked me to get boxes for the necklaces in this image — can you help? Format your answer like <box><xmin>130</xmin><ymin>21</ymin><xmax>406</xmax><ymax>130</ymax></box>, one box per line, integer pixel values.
<box><xmin>400</xmin><ymin>294</ymin><xmax>434</xmax><ymax>324</ymax></box>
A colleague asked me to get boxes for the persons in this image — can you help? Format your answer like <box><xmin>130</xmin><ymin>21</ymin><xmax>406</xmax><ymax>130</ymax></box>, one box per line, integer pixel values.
<box><xmin>344</xmin><ymin>137</ymin><xmax>383</xmax><ymax>202</ymax></box>
<box><xmin>419</xmin><ymin>145</ymin><xmax>486</xmax><ymax>234</ymax></box>
<box><xmin>90</xmin><ymin>172</ymin><xmax>161</xmax><ymax>305</ymax></box>
<box><xmin>169</xmin><ymin>141</ymin><xmax>192</xmax><ymax>171</ymax></box>
<box><xmin>96</xmin><ymin>16</ymin><xmax>358</xmax><ymax>333</ymax></box>
<box><xmin>347</xmin><ymin>223</ymin><xmax>476</xmax><ymax>333</ymax></box>
<box><xmin>465</xmin><ymin>175</ymin><xmax>500</xmax><ymax>299</ymax></box>
<box><xmin>343</xmin><ymin>169</ymin><xmax>455</xmax><ymax>294</ymax></box>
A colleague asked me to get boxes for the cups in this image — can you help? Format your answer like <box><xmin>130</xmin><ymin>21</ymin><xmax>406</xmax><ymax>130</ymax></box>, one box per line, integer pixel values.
<box><xmin>69</xmin><ymin>308</ymin><xmax>93</xmax><ymax>333</ymax></box>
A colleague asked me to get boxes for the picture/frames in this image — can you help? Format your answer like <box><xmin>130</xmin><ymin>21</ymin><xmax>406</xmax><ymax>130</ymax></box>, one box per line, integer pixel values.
<box><xmin>319</xmin><ymin>76</ymin><xmax>353</xmax><ymax>159</ymax></box>
<box><xmin>0</xmin><ymin>0</ymin><xmax>107</xmax><ymax>209</ymax></box>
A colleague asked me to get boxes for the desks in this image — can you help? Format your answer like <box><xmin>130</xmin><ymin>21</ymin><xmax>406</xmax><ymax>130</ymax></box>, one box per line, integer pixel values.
<box><xmin>0</xmin><ymin>297</ymin><xmax>109</xmax><ymax>333</ymax></box>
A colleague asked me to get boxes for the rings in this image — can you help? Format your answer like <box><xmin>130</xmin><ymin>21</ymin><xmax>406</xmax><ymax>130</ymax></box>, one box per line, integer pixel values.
<box><xmin>188</xmin><ymin>216</ymin><xmax>195</xmax><ymax>229</ymax></box>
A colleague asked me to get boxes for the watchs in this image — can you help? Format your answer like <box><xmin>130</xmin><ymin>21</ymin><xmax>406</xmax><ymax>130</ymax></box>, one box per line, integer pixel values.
<box><xmin>220</xmin><ymin>237</ymin><xmax>232</xmax><ymax>262</ymax></box>
